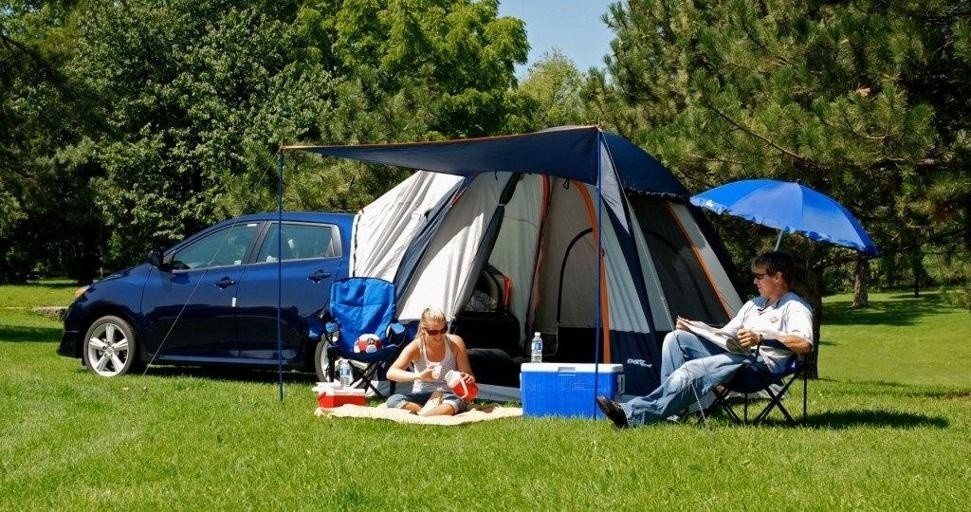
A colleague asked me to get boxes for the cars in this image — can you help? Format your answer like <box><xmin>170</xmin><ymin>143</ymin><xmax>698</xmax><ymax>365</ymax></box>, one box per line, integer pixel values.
<box><xmin>56</xmin><ymin>210</ymin><xmax>512</xmax><ymax>381</ymax></box>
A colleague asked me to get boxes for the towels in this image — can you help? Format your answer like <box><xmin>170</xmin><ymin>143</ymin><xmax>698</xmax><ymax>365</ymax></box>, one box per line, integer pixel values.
<box><xmin>320</xmin><ymin>399</ymin><xmax>523</xmax><ymax>428</ymax></box>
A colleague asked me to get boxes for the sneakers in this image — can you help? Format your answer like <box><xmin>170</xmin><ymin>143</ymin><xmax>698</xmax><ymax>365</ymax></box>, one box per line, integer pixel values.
<box><xmin>596</xmin><ymin>393</ymin><xmax>629</xmax><ymax>429</ymax></box>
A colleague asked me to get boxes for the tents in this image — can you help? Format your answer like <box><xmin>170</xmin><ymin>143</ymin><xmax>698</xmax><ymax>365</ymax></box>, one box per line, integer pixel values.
<box><xmin>284</xmin><ymin>126</ymin><xmax>786</xmax><ymax>413</ymax></box>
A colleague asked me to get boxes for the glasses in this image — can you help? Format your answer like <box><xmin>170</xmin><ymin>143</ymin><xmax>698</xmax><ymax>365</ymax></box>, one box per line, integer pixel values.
<box><xmin>753</xmin><ymin>271</ymin><xmax>769</xmax><ymax>281</ymax></box>
<box><xmin>422</xmin><ymin>325</ymin><xmax>448</xmax><ymax>335</ymax></box>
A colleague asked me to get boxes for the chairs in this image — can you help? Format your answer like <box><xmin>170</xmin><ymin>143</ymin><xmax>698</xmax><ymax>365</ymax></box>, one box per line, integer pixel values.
<box><xmin>318</xmin><ymin>275</ymin><xmax>409</xmax><ymax>402</ymax></box>
<box><xmin>693</xmin><ymin>301</ymin><xmax>819</xmax><ymax>424</ymax></box>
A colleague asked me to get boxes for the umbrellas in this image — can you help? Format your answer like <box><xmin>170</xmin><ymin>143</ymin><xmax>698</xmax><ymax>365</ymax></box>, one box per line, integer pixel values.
<box><xmin>688</xmin><ymin>177</ymin><xmax>881</xmax><ymax>258</ymax></box>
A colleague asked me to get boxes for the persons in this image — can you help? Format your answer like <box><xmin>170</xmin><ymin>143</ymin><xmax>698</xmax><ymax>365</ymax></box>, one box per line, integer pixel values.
<box><xmin>597</xmin><ymin>252</ymin><xmax>814</xmax><ymax>429</ymax></box>
<box><xmin>386</xmin><ymin>306</ymin><xmax>477</xmax><ymax>417</ymax></box>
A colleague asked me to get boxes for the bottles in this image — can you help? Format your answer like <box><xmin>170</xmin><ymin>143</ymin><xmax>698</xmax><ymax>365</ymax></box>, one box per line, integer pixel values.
<box><xmin>530</xmin><ymin>332</ymin><xmax>544</xmax><ymax>365</ymax></box>
<box><xmin>339</xmin><ymin>358</ymin><xmax>353</xmax><ymax>392</ymax></box>
<box><xmin>444</xmin><ymin>369</ymin><xmax>479</xmax><ymax>403</ymax></box>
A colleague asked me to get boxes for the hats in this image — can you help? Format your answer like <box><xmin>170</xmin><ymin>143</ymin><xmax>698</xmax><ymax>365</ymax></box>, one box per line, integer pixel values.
<box><xmin>353</xmin><ymin>332</ymin><xmax>384</xmax><ymax>355</ymax></box>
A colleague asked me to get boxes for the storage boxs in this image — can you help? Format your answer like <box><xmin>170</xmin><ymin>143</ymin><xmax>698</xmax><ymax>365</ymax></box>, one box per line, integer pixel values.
<box><xmin>316</xmin><ymin>387</ymin><xmax>366</xmax><ymax>410</ymax></box>
<box><xmin>518</xmin><ymin>360</ymin><xmax>626</xmax><ymax>422</ymax></box>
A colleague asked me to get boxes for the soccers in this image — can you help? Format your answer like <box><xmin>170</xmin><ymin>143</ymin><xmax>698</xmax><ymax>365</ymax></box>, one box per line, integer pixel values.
<box><xmin>358</xmin><ymin>334</ymin><xmax>383</xmax><ymax>353</ymax></box>
